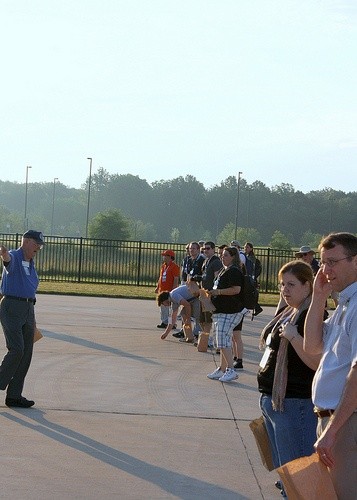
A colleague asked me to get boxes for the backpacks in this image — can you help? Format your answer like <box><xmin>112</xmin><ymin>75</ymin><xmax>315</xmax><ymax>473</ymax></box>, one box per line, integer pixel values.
<box><xmin>254</xmin><ymin>259</ymin><xmax>262</xmax><ymax>277</ymax></box>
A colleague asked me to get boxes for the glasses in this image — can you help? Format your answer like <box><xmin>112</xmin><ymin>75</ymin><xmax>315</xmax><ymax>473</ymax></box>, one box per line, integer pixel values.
<box><xmin>300</xmin><ymin>252</ymin><xmax>309</xmax><ymax>258</ymax></box>
<box><xmin>318</xmin><ymin>253</ymin><xmax>357</xmax><ymax>269</ymax></box>
<box><xmin>29</xmin><ymin>240</ymin><xmax>43</xmax><ymax>249</ymax></box>
<box><xmin>204</xmin><ymin>247</ymin><xmax>212</xmax><ymax>251</ymax></box>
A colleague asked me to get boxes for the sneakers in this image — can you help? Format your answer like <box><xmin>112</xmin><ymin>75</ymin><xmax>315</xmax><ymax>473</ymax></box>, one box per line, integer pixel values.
<box><xmin>219</xmin><ymin>370</ymin><xmax>238</xmax><ymax>381</ymax></box>
<box><xmin>207</xmin><ymin>367</ymin><xmax>225</xmax><ymax>380</ymax></box>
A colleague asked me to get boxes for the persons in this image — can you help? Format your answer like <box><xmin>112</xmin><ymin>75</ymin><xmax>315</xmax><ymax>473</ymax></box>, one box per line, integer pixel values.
<box><xmin>256</xmin><ymin>260</ymin><xmax>330</xmax><ymax>495</ymax></box>
<box><xmin>0</xmin><ymin>229</ymin><xmax>49</xmax><ymax>408</ymax></box>
<box><xmin>155</xmin><ymin>238</ymin><xmax>263</xmax><ymax>370</ymax></box>
<box><xmin>302</xmin><ymin>231</ymin><xmax>357</xmax><ymax>500</ymax></box>
<box><xmin>294</xmin><ymin>245</ymin><xmax>328</xmax><ymax>308</ymax></box>
<box><xmin>206</xmin><ymin>246</ymin><xmax>247</xmax><ymax>381</ymax></box>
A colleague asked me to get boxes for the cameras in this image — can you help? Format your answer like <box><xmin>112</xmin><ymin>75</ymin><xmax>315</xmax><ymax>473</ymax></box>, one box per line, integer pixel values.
<box><xmin>191</xmin><ymin>275</ymin><xmax>202</xmax><ymax>282</ymax></box>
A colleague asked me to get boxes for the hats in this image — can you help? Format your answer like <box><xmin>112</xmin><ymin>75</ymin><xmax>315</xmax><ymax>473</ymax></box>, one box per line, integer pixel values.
<box><xmin>198</xmin><ymin>240</ymin><xmax>205</xmax><ymax>244</ymax></box>
<box><xmin>160</xmin><ymin>250</ymin><xmax>175</xmax><ymax>258</ymax></box>
<box><xmin>295</xmin><ymin>245</ymin><xmax>315</xmax><ymax>258</ymax></box>
<box><xmin>23</xmin><ymin>229</ymin><xmax>45</xmax><ymax>246</ymax></box>
<box><xmin>231</xmin><ymin>241</ymin><xmax>240</xmax><ymax>248</ymax></box>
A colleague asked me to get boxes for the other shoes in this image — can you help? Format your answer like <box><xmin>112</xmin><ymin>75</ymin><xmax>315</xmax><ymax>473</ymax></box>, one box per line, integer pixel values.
<box><xmin>274</xmin><ymin>480</ymin><xmax>281</xmax><ymax>489</ymax></box>
<box><xmin>178</xmin><ymin>338</ymin><xmax>186</xmax><ymax>341</ymax></box>
<box><xmin>232</xmin><ymin>361</ymin><xmax>244</xmax><ymax>370</ymax></box>
<box><xmin>173</xmin><ymin>326</ymin><xmax>177</xmax><ymax>329</ymax></box>
<box><xmin>4</xmin><ymin>397</ymin><xmax>36</xmax><ymax>408</ymax></box>
<box><xmin>207</xmin><ymin>344</ymin><xmax>213</xmax><ymax>349</ymax></box>
<box><xmin>194</xmin><ymin>342</ymin><xmax>198</xmax><ymax>346</ymax></box>
<box><xmin>280</xmin><ymin>490</ymin><xmax>284</xmax><ymax>494</ymax></box>
<box><xmin>216</xmin><ymin>349</ymin><xmax>220</xmax><ymax>354</ymax></box>
<box><xmin>157</xmin><ymin>323</ymin><xmax>168</xmax><ymax>328</ymax></box>
<box><xmin>172</xmin><ymin>332</ymin><xmax>184</xmax><ymax>338</ymax></box>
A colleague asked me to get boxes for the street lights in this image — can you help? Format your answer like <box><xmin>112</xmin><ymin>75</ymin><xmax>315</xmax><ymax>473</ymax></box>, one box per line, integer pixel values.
<box><xmin>86</xmin><ymin>157</ymin><xmax>93</xmax><ymax>236</ymax></box>
<box><xmin>245</xmin><ymin>187</ymin><xmax>255</xmax><ymax>236</ymax></box>
<box><xmin>234</xmin><ymin>170</ymin><xmax>243</xmax><ymax>242</ymax></box>
<box><xmin>25</xmin><ymin>166</ymin><xmax>33</xmax><ymax>232</ymax></box>
<box><xmin>50</xmin><ymin>178</ymin><xmax>58</xmax><ymax>234</ymax></box>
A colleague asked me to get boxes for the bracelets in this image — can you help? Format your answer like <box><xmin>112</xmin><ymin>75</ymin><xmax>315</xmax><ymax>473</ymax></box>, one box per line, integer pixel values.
<box><xmin>289</xmin><ymin>333</ymin><xmax>298</xmax><ymax>342</ymax></box>
<box><xmin>218</xmin><ymin>288</ymin><xmax>220</xmax><ymax>295</ymax></box>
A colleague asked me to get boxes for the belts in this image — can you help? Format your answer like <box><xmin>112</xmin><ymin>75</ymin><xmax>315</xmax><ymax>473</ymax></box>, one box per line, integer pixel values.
<box><xmin>315</xmin><ymin>409</ymin><xmax>336</xmax><ymax>418</ymax></box>
<box><xmin>4</xmin><ymin>295</ymin><xmax>34</xmax><ymax>302</ymax></box>
